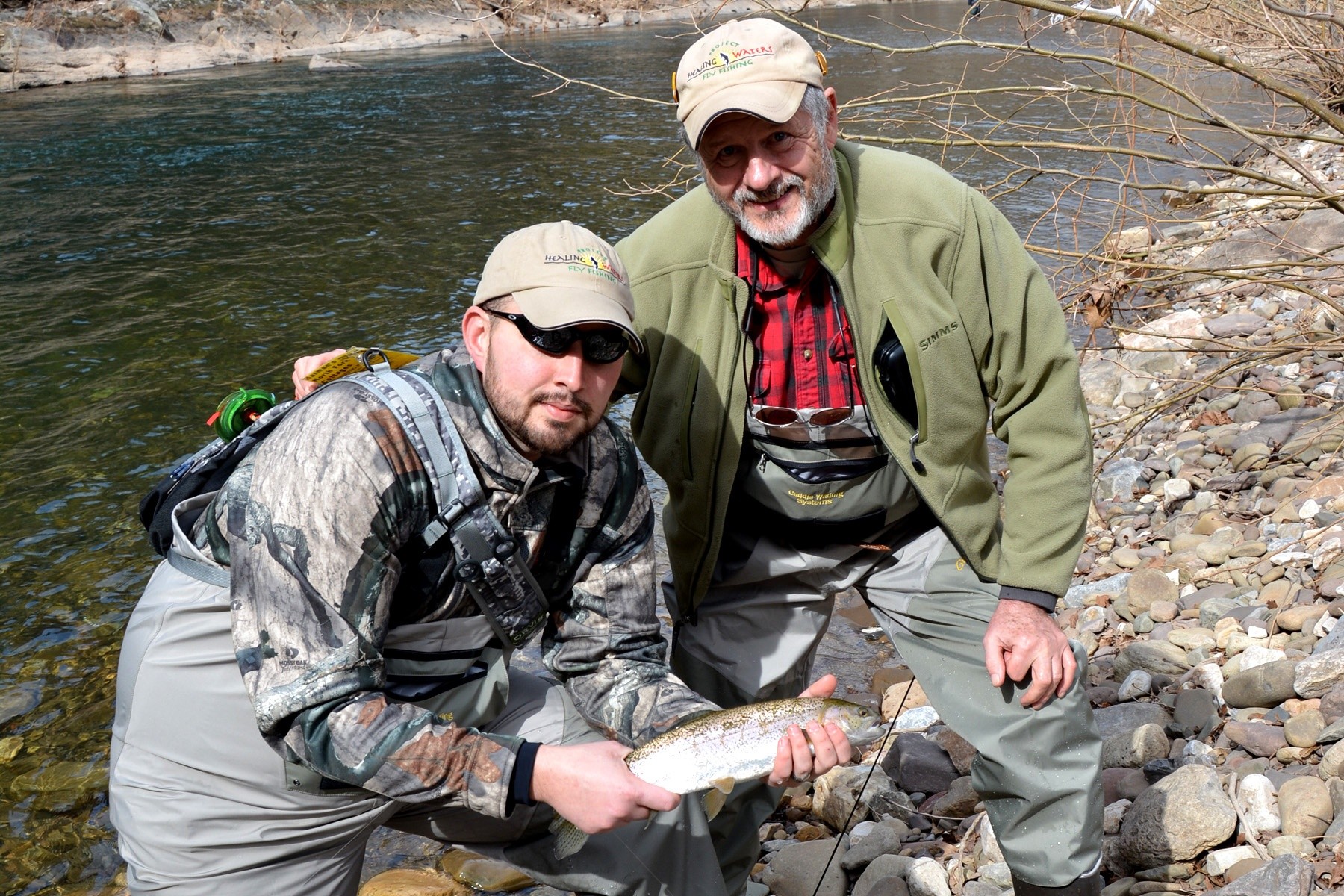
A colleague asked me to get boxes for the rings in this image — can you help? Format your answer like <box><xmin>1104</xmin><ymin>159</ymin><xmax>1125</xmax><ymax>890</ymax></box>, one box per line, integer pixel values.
<box><xmin>792</xmin><ymin>772</ymin><xmax>811</xmax><ymax>784</ymax></box>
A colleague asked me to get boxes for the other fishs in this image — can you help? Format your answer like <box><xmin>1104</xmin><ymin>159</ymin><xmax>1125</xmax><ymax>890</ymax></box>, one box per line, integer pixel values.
<box><xmin>545</xmin><ymin>697</ymin><xmax>886</xmax><ymax>859</ymax></box>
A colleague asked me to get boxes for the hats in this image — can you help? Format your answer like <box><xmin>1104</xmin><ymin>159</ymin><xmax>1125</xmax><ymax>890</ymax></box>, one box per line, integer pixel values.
<box><xmin>473</xmin><ymin>219</ymin><xmax>644</xmax><ymax>356</ymax></box>
<box><xmin>676</xmin><ymin>19</ymin><xmax>825</xmax><ymax>152</ymax></box>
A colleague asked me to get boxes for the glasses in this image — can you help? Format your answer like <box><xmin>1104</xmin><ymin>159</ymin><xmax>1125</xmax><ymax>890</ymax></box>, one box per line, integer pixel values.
<box><xmin>742</xmin><ymin>277</ymin><xmax>855</xmax><ymax>428</ymax></box>
<box><xmin>482</xmin><ymin>308</ymin><xmax>631</xmax><ymax>364</ymax></box>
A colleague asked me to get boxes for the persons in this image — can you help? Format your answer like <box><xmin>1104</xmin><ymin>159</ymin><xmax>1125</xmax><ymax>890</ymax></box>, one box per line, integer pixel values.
<box><xmin>103</xmin><ymin>219</ymin><xmax>858</xmax><ymax>895</ymax></box>
<box><xmin>291</xmin><ymin>15</ymin><xmax>1106</xmax><ymax>895</ymax></box>
